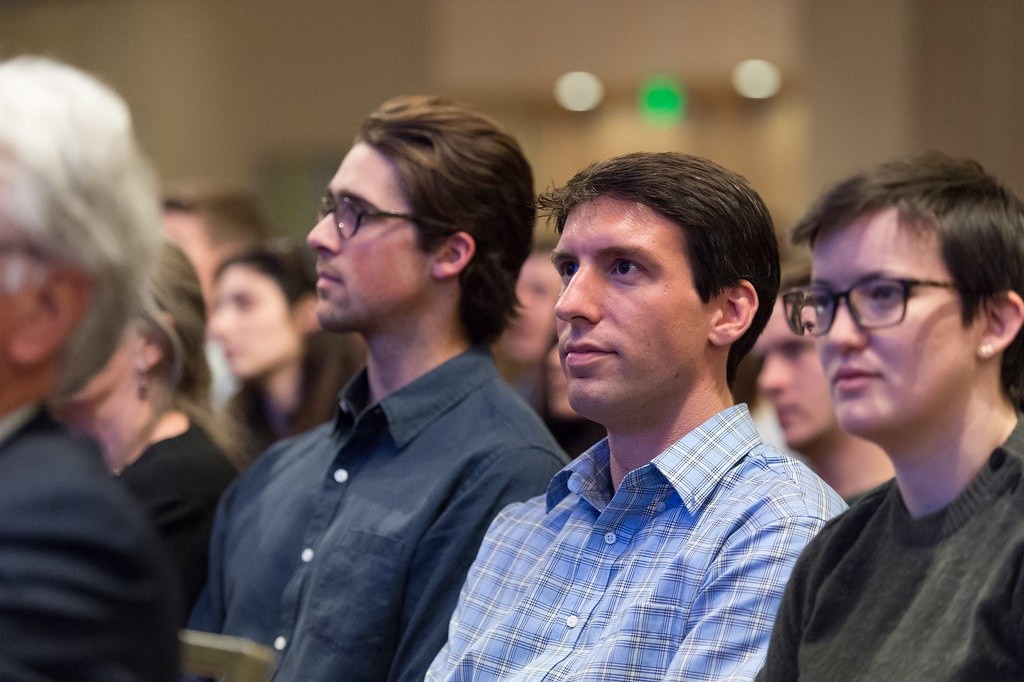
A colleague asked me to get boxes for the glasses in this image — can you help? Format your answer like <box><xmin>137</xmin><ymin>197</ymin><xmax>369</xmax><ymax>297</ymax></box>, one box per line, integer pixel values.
<box><xmin>316</xmin><ymin>194</ymin><xmax>463</xmax><ymax>239</ymax></box>
<box><xmin>783</xmin><ymin>270</ymin><xmax>961</xmax><ymax>339</ymax></box>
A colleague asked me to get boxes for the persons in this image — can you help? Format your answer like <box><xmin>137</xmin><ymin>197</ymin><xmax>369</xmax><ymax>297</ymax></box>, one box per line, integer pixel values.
<box><xmin>755</xmin><ymin>267</ymin><xmax>896</xmax><ymax>507</ymax></box>
<box><xmin>0</xmin><ymin>57</ymin><xmax>181</xmax><ymax>682</ymax></box>
<box><xmin>184</xmin><ymin>95</ymin><xmax>572</xmax><ymax>682</ymax></box>
<box><xmin>425</xmin><ymin>151</ymin><xmax>848</xmax><ymax>681</ymax></box>
<box><xmin>62</xmin><ymin>190</ymin><xmax>271</xmax><ymax>622</ymax></box>
<box><xmin>755</xmin><ymin>152</ymin><xmax>1024</xmax><ymax>682</ymax></box>
